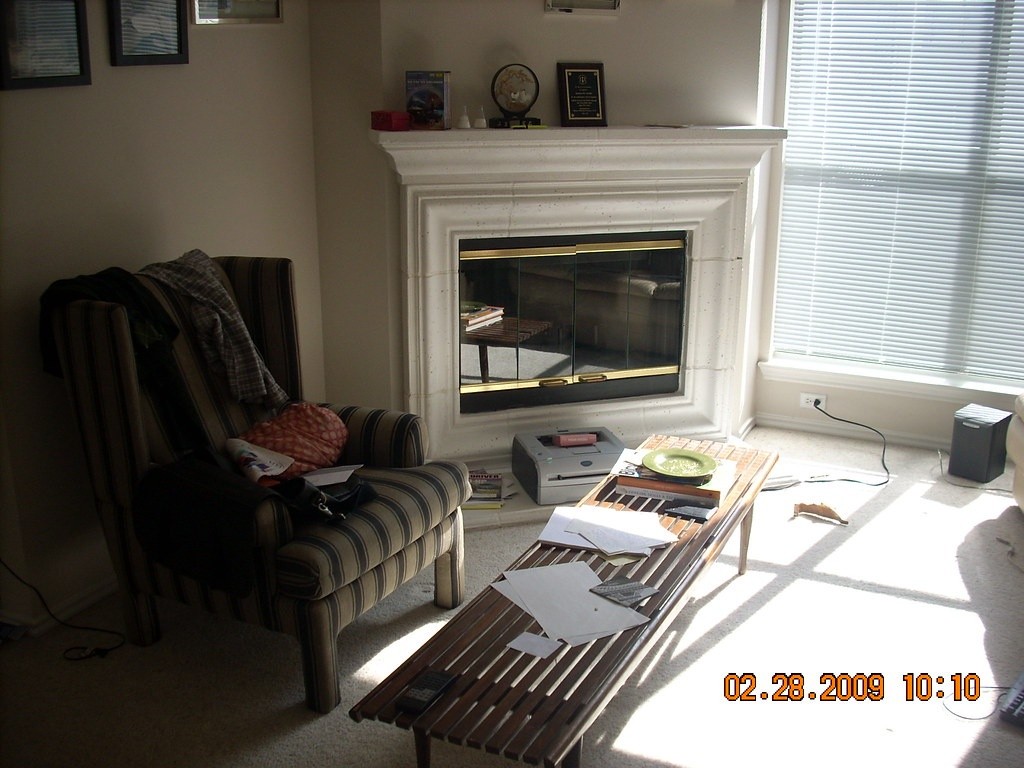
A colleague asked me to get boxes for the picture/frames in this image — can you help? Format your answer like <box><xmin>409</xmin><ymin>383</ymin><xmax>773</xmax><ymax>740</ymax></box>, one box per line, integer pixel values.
<box><xmin>108</xmin><ymin>0</ymin><xmax>189</xmax><ymax>67</ymax></box>
<box><xmin>0</xmin><ymin>0</ymin><xmax>92</xmax><ymax>90</ymax></box>
<box><xmin>190</xmin><ymin>0</ymin><xmax>284</xmax><ymax>25</ymax></box>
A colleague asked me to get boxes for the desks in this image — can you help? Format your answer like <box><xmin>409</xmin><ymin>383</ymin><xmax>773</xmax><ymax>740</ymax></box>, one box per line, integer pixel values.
<box><xmin>349</xmin><ymin>432</ymin><xmax>778</xmax><ymax>768</ymax></box>
<box><xmin>460</xmin><ymin>318</ymin><xmax>553</xmax><ymax>384</ymax></box>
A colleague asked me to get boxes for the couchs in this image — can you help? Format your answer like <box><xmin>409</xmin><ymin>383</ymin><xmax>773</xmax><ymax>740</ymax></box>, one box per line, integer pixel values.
<box><xmin>510</xmin><ymin>257</ymin><xmax>685</xmax><ymax>357</ymax></box>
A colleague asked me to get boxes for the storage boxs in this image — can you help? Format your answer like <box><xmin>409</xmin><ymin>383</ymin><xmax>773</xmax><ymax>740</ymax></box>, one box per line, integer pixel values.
<box><xmin>404</xmin><ymin>70</ymin><xmax>452</xmax><ymax>130</ymax></box>
<box><xmin>370</xmin><ymin>110</ymin><xmax>411</xmax><ymax>132</ymax></box>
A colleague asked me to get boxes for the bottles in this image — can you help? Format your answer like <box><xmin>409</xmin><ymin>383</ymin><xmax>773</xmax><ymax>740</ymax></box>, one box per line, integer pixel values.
<box><xmin>474</xmin><ymin>105</ymin><xmax>487</xmax><ymax>128</ymax></box>
<box><xmin>457</xmin><ymin>105</ymin><xmax>470</xmax><ymax>128</ymax></box>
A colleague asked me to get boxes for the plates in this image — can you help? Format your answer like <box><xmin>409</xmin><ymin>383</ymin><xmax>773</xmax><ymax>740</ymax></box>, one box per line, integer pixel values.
<box><xmin>460</xmin><ymin>301</ymin><xmax>486</xmax><ymax>312</ymax></box>
<box><xmin>642</xmin><ymin>448</ymin><xmax>716</xmax><ymax>477</ymax></box>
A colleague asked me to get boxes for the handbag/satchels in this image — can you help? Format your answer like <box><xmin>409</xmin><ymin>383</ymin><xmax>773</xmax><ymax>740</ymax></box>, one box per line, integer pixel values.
<box><xmin>275</xmin><ymin>473</ymin><xmax>378</xmax><ymax>532</ymax></box>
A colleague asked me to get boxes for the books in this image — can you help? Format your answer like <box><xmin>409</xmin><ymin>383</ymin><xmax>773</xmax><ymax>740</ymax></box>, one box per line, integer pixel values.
<box><xmin>461</xmin><ymin>469</ymin><xmax>518</xmax><ymax>510</ymax></box>
<box><xmin>461</xmin><ymin>306</ymin><xmax>505</xmax><ymax>332</ymax></box>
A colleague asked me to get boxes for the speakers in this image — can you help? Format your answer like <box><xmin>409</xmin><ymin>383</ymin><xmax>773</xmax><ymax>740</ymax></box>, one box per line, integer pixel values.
<box><xmin>949</xmin><ymin>402</ymin><xmax>1013</xmax><ymax>483</ymax></box>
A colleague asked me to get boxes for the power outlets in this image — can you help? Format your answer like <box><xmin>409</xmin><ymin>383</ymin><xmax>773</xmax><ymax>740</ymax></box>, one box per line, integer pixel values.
<box><xmin>801</xmin><ymin>393</ymin><xmax>826</xmax><ymax>411</ymax></box>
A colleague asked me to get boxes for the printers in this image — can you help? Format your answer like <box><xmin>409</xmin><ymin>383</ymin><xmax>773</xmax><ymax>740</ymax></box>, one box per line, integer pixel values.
<box><xmin>511</xmin><ymin>426</ymin><xmax>626</xmax><ymax>505</ymax></box>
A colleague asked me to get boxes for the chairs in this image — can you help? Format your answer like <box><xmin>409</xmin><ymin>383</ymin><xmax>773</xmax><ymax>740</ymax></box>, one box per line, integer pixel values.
<box><xmin>61</xmin><ymin>253</ymin><xmax>472</xmax><ymax>714</ymax></box>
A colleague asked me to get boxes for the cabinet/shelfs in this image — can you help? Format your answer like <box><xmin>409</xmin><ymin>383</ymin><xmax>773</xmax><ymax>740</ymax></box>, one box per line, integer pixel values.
<box><xmin>458</xmin><ymin>229</ymin><xmax>688</xmax><ymax>415</ymax></box>
